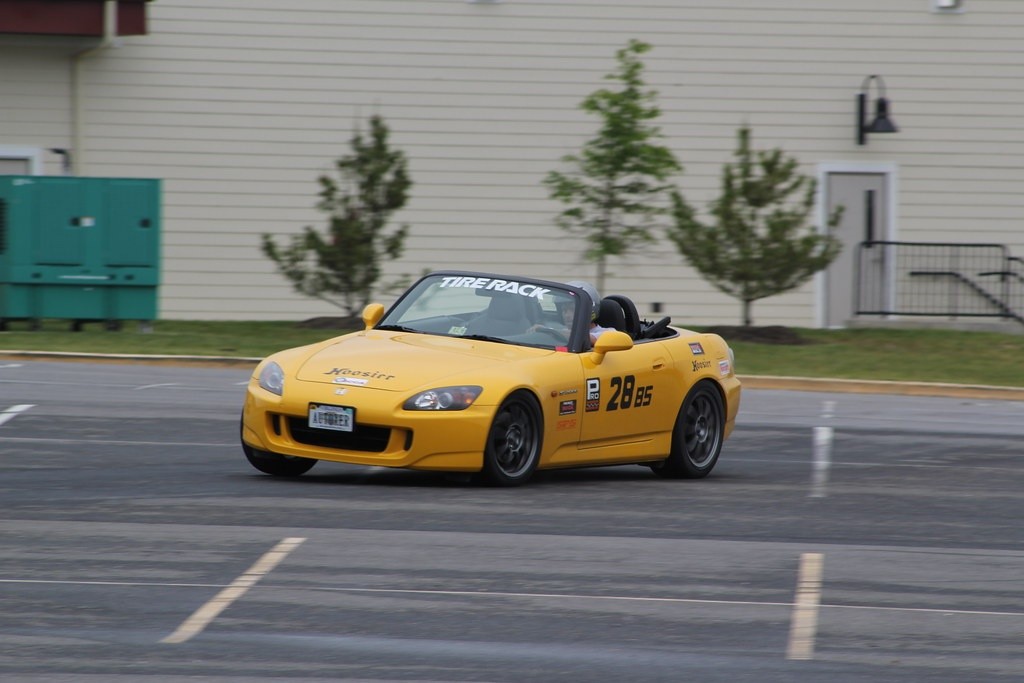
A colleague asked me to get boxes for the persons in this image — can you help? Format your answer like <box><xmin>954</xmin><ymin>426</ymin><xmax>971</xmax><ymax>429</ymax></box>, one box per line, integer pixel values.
<box><xmin>526</xmin><ymin>281</ymin><xmax>615</xmax><ymax>346</ymax></box>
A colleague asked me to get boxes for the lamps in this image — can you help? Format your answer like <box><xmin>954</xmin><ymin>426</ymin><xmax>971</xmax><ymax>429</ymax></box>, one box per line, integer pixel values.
<box><xmin>857</xmin><ymin>75</ymin><xmax>899</xmax><ymax>144</ymax></box>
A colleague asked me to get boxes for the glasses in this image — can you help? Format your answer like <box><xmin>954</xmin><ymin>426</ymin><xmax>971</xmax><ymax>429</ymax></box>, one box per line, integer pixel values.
<box><xmin>562</xmin><ymin>306</ymin><xmax>574</xmax><ymax>314</ymax></box>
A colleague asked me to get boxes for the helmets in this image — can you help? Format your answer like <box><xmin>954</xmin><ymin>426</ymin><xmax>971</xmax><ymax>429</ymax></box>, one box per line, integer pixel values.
<box><xmin>543</xmin><ymin>281</ymin><xmax>601</xmax><ymax>321</ymax></box>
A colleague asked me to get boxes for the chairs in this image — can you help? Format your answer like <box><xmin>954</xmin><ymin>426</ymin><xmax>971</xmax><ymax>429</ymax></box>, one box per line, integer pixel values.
<box><xmin>470</xmin><ymin>293</ymin><xmax>532</xmax><ymax>336</ymax></box>
<box><xmin>595</xmin><ymin>300</ymin><xmax>628</xmax><ymax>333</ymax></box>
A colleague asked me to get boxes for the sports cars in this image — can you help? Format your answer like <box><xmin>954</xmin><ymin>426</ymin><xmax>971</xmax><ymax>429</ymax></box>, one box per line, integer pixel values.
<box><xmin>239</xmin><ymin>269</ymin><xmax>744</xmax><ymax>488</ymax></box>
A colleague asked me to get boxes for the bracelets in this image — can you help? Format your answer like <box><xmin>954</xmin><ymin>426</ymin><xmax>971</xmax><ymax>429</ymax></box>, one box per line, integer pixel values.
<box><xmin>550</xmin><ymin>328</ymin><xmax>554</xmax><ymax>329</ymax></box>
